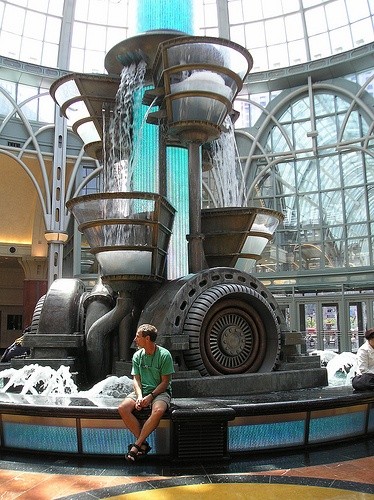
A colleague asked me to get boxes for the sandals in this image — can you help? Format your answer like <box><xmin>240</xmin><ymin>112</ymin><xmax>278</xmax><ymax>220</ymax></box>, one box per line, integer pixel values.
<box><xmin>134</xmin><ymin>441</ymin><xmax>152</xmax><ymax>460</ymax></box>
<box><xmin>124</xmin><ymin>443</ymin><xmax>141</xmax><ymax>463</ymax></box>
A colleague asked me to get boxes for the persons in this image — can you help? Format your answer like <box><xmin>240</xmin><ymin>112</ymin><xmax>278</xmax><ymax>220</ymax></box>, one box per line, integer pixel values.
<box><xmin>118</xmin><ymin>324</ymin><xmax>175</xmax><ymax>462</ymax></box>
<box><xmin>357</xmin><ymin>328</ymin><xmax>374</xmax><ymax>375</ymax></box>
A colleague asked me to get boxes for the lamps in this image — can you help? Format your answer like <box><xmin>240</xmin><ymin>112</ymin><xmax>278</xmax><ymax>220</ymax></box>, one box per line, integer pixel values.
<box><xmin>45</xmin><ymin>231</ymin><xmax>68</xmax><ymax>244</ymax></box>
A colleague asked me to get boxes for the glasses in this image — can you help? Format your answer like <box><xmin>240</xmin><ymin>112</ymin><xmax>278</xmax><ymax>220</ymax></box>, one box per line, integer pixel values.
<box><xmin>140</xmin><ymin>356</ymin><xmax>152</xmax><ymax>368</ymax></box>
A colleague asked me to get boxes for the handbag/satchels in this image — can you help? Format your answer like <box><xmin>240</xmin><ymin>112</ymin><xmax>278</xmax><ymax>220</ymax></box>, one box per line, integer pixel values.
<box><xmin>352</xmin><ymin>373</ymin><xmax>374</xmax><ymax>391</ymax></box>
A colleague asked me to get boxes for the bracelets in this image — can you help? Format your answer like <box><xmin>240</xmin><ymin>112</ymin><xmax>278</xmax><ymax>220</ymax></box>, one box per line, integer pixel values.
<box><xmin>150</xmin><ymin>392</ymin><xmax>155</xmax><ymax>399</ymax></box>
